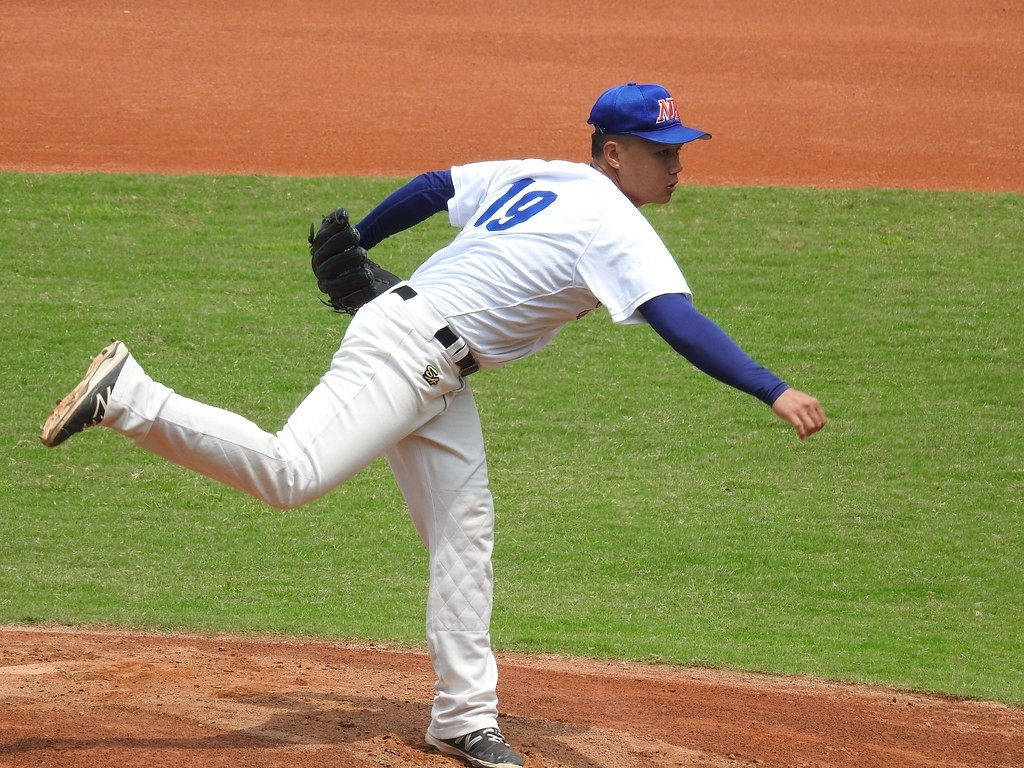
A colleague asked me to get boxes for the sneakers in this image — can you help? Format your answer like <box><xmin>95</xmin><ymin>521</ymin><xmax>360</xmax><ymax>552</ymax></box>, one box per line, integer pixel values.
<box><xmin>38</xmin><ymin>337</ymin><xmax>129</xmax><ymax>448</ymax></box>
<box><xmin>425</xmin><ymin>726</ymin><xmax>524</xmax><ymax>768</ymax></box>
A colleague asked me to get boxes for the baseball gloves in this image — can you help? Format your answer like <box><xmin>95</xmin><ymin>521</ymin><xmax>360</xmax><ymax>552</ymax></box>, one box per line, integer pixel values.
<box><xmin>308</xmin><ymin>206</ymin><xmax>401</xmax><ymax>314</ymax></box>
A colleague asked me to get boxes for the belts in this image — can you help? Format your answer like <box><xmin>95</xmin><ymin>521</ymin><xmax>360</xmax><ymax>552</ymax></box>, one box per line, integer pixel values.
<box><xmin>391</xmin><ymin>284</ymin><xmax>480</xmax><ymax>378</ymax></box>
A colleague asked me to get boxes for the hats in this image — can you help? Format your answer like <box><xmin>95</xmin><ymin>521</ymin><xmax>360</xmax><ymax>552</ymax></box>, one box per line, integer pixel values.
<box><xmin>587</xmin><ymin>82</ymin><xmax>712</xmax><ymax>144</ymax></box>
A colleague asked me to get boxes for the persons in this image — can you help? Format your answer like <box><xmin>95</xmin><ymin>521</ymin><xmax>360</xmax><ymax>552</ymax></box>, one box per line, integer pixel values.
<box><xmin>39</xmin><ymin>83</ymin><xmax>827</xmax><ymax>768</ymax></box>
